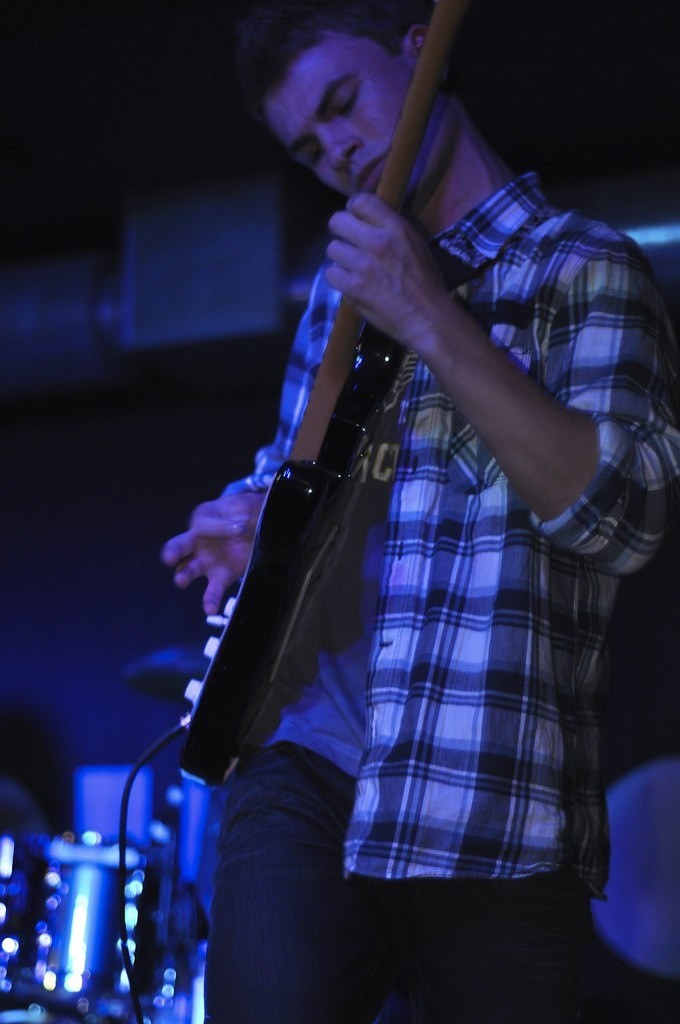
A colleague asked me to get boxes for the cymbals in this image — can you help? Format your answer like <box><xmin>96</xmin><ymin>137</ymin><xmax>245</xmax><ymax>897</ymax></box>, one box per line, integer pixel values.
<box><xmin>121</xmin><ymin>644</ymin><xmax>209</xmax><ymax>704</ymax></box>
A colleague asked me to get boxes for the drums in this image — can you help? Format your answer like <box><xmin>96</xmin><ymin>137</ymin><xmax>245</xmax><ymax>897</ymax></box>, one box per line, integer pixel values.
<box><xmin>588</xmin><ymin>754</ymin><xmax>679</xmax><ymax>986</ymax></box>
<box><xmin>1</xmin><ymin>834</ymin><xmax>163</xmax><ymax>1022</ymax></box>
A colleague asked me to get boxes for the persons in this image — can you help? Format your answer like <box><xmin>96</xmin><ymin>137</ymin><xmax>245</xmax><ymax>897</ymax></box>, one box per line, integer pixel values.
<box><xmin>161</xmin><ymin>0</ymin><xmax>679</xmax><ymax>1024</ymax></box>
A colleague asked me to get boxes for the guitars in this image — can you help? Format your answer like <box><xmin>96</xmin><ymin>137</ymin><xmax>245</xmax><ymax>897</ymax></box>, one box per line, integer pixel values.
<box><xmin>176</xmin><ymin>0</ymin><xmax>477</xmax><ymax>791</ymax></box>
